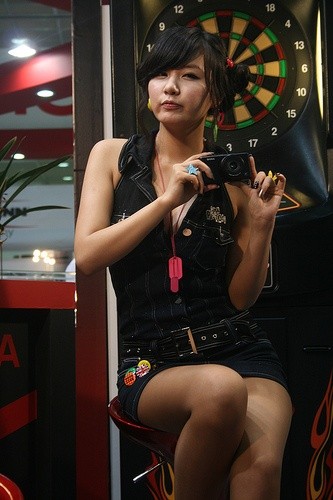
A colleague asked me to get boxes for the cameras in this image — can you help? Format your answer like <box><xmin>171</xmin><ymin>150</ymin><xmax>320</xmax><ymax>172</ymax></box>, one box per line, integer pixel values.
<box><xmin>200</xmin><ymin>152</ymin><xmax>251</xmax><ymax>186</ymax></box>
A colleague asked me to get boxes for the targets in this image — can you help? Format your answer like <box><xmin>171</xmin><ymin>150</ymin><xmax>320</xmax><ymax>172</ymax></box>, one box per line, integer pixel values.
<box><xmin>139</xmin><ymin>0</ymin><xmax>312</xmax><ymax>154</ymax></box>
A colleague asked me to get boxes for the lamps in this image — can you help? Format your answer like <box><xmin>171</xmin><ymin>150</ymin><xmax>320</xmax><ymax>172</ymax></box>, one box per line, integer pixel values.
<box><xmin>7</xmin><ymin>36</ymin><xmax>37</xmax><ymax>59</ymax></box>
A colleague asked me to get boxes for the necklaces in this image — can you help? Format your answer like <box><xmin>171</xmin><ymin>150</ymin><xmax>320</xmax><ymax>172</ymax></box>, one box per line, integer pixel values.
<box><xmin>150</xmin><ymin>133</ymin><xmax>209</xmax><ymax>294</ymax></box>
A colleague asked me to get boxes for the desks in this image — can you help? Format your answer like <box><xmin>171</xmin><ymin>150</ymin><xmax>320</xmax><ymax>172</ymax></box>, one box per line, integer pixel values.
<box><xmin>0</xmin><ymin>252</ymin><xmax>77</xmax><ymax>309</ymax></box>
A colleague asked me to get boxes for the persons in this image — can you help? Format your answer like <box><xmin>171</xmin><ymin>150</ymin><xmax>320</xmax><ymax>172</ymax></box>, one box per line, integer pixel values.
<box><xmin>74</xmin><ymin>28</ymin><xmax>294</xmax><ymax>500</ymax></box>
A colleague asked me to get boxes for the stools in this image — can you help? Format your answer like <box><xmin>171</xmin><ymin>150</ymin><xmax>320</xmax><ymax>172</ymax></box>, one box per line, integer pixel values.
<box><xmin>108</xmin><ymin>394</ymin><xmax>179</xmax><ymax>500</ymax></box>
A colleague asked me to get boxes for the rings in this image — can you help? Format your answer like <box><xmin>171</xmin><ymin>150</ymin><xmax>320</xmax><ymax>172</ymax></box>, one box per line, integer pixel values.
<box><xmin>184</xmin><ymin>164</ymin><xmax>199</xmax><ymax>175</ymax></box>
<box><xmin>268</xmin><ymin>170</ymin><xmax>278</xmax><ymax>186</ymax></box>
